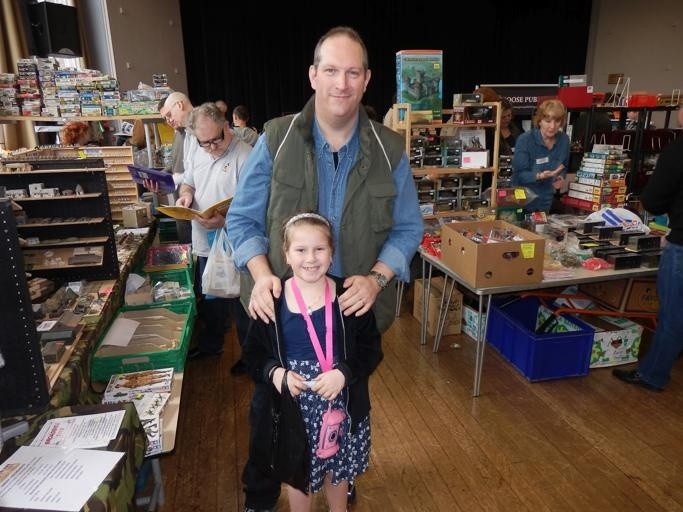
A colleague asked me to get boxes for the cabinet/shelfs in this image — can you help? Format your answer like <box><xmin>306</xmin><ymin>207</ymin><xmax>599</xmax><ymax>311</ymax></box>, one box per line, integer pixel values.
<box><xmin>0</xmin><ymin>111</ymin><xmax>177</xmax><ymax>212</ymax></box>
<box><xmin>32</xmin><ymin>147</ymin><xmax>137</xmax><ymax>221</ymax></box>
<box><xmin>567</xmin><ymin>105</ymin><xmax>681</xmax><ymax>189</ymax></box>
<box><xmin>0</xmin><ymin>161</ymin><xmax>117</xmax><ymax>280</ymax></box>
<box><xmin>392</xmin><ymin>102</ymin><xmax>496</xmax><ymax>218</ymax></box>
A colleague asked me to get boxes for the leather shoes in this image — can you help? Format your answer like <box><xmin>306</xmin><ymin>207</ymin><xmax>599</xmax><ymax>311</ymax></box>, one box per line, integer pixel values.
<box><xmin>612</xmin><ymin>369</ymin><xmax>662</xmax><ymax>391</ymax></box>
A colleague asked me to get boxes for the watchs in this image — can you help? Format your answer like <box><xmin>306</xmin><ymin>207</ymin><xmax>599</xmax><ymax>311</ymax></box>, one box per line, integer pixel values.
<box><xmin>369</xmin><ymin>269</ymin><xmax>389</xmax><ymax>293</ymax></box>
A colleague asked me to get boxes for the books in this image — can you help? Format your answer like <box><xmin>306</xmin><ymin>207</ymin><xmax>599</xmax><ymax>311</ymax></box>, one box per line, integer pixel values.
<box><xmin>0</xmin><ymin>50</ymin><xmax>184</xmax><ymax>511</ymax></box>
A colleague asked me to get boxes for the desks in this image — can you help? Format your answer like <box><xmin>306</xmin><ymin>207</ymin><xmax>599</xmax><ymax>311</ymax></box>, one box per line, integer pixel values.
<box><xmin>414</xmin><ymin>243</ymin><xmax>661</xmax><ymax>397</ymax></box>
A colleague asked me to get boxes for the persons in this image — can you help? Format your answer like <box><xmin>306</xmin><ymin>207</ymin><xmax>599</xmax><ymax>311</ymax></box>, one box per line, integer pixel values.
<box><xmin>237</xmin><ymin>206</ymin><xmax>388</xmax><ymax>511</ymax></box>
<box><xmin>511</xmin><ymin>100</ymin><xmax>571</xmax><ymax>216</ymax></box>
<box><xmin>221</xmin><ymin>25</ymin><xmax>427</xmax><ymax>512</ymax></box>
<box><xmin>611</xmin><ymin>91</ymin><xmax>682</xmax><ymax>396</ymax></box>
<box><xmin>439</xmin><ymin>86</ymin><xmax>523</xmax><ymax>158</ymax></box>
<box><xmin>143</xmin><ymin>91</ymin><xmax>260</xmax><ymax>246</ymax></box>
<box><xmin>175</xmin><ymin>101</ymin><xmax>255</xmax><ymax>378</ymax></box>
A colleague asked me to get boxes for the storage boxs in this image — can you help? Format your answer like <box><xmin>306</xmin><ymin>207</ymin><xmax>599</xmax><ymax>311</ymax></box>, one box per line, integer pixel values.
<box><xmin>441</xmin><ymin>219</ymin><xmax>544</xmax><ymax>288</ymax></box>
<box><xmin>412</xmin><ymin>278</ymin><xmax>462</xmax><ymax>336</ymax></box>
<box><xmin>459</xmin><ymin>296</ymin><xmax>489</xmax><ymax>341</ymax></box>
<box><xmin>535</xmin><ymin>300</ymin><xmax>643</xmax><ymax>368</ymax></box>
<box><xmin>489</xmin><ymin>299</ymin><xmax>593</xmax><ymax>381</ymax></box>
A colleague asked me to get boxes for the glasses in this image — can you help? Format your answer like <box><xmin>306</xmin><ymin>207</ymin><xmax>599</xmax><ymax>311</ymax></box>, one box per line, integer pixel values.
<box><xmin>196</xmin><ymin>129</ymin><xmax>224</xmax><ymax>147</ymax></box>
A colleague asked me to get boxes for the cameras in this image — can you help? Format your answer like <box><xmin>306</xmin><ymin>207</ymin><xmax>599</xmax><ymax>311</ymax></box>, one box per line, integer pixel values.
<box><xmin>323</xmin><ymin>423</ymin><xmax>340</xmax><ymax>450</ymax></box>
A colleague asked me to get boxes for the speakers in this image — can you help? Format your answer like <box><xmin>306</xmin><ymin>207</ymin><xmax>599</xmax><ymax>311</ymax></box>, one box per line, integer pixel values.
<box><xmin>29</xmin><ymin>2</ymin><xmax>82</xmax><ymax>58</ymax></box>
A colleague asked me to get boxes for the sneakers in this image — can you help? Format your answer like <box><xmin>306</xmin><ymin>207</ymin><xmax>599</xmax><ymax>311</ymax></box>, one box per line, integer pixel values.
<box><xmin>187</xmin><ymin>345</ymin><xmax>223</xmax><ymax>358</ymax></box>
<box><xmin>231</xmin><ymin>360</ymin><xmax>249</xmax><ymax>375</ymax></box>
